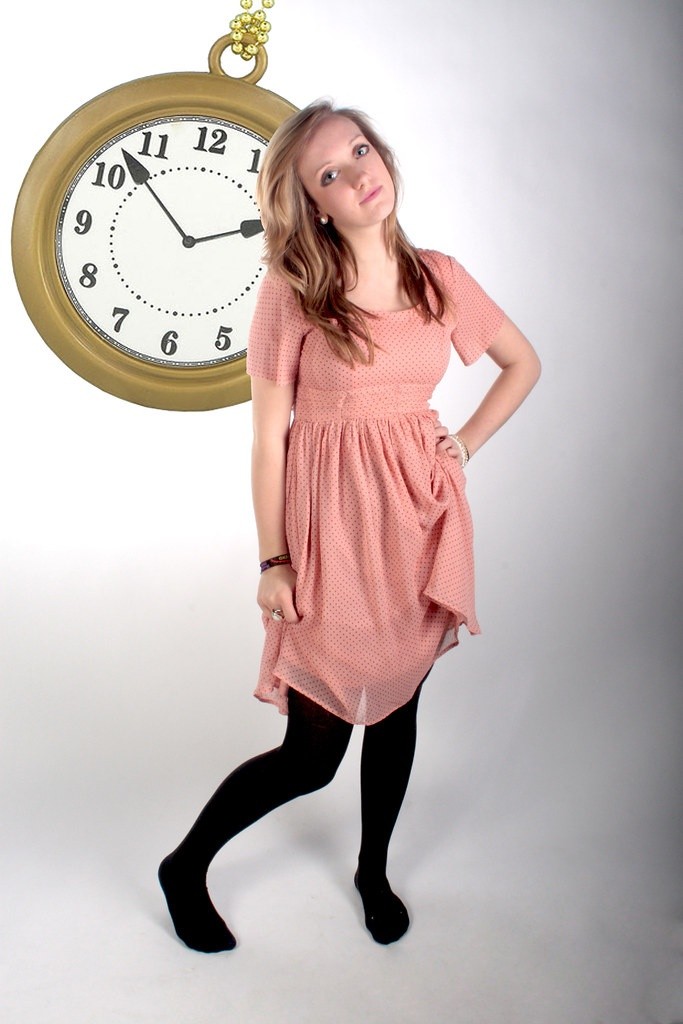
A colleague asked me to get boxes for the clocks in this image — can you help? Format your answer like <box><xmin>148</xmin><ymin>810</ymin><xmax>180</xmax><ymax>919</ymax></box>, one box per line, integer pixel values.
<box><xmin>13</xmin><ymin>32</ymin><xmax>302</xmax><ymax>408</ymax></box>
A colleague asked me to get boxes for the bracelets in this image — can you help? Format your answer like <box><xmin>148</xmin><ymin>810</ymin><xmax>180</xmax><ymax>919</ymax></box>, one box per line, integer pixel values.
<box><xmin>450</xmin><ymin>434</ymin><xmax>470</xmax><ymax>467</ymax></box>
<box><xmin>260</xmin><ymin>554</ymin><xmax>290</xmax><ymax>573</ymax></box>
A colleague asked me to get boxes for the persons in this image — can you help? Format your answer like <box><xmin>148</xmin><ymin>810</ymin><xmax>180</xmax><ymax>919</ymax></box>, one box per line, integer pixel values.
<box><xmin>158</xmin><ymin>105</ymin><xmax>543</xmax><ymax>954</ymax></box>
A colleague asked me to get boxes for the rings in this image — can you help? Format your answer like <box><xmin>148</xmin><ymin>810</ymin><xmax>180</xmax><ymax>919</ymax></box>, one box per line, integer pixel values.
<box><xmin>274</xmin><ymin>610</ymin><xmax>281</xmax><ymax>615</ymax></box>
